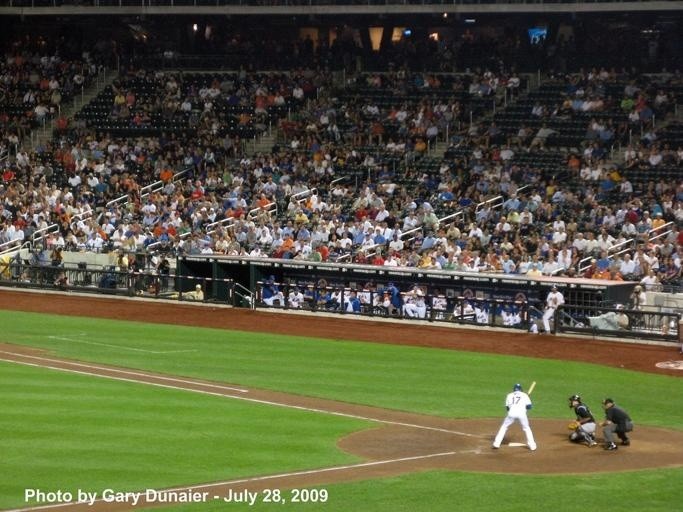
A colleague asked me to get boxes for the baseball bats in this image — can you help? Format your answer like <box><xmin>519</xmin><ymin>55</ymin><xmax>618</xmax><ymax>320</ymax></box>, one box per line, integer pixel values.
<box><xmin>527</xmin><ymin>382</ymin><xmax>536</xmax><ymax>395</ymax></box>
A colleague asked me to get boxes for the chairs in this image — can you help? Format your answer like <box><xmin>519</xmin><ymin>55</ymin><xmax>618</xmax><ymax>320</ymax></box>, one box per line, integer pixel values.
<box><xmin>1</xmin><ymin>26</ymin><xmax>682</xmax><ymax>336</ymax></box>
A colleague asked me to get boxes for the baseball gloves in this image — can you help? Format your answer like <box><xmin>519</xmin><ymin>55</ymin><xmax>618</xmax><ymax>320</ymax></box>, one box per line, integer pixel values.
<box><xmin>568</xmin><ymin>420</ymin><xmax>580</xmax><ymax>430</ymax></box>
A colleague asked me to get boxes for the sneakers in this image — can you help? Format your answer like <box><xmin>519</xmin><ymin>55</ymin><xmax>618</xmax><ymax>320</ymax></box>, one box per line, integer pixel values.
<box><xmin>589</xmin><ymin>432</ymin><xmax>595</xmax><ymax>440</ymax></box>
<box><xmin>587</xmin><ymin>440</ymin><xmax>597</xmax><ymax>448</ymax></box>
<box><xmin>604</xmin><ymin>441</ymin><xmax>617</xmax><ymax>450</ymax></box>
<box><xmin>620</xmin><ymin>438</ymin><xmax>630</xmax><ymax>445</ymax></box>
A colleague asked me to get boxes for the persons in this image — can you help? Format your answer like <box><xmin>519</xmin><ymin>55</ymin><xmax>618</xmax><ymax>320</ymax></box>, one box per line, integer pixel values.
<box><xmin>1</xmin><ymin>20</ymin><xmax>683</xmax><ymax>340</ymax></box>
<box><xmin>488</xmin><ymin>384</ymin><xmax>537</xmax><ymax>451</ymax></box>
<box><xmin>566</xmin><ymin>395</ymin><xmax>598</xmax><ymax>448</ymax></box>
<box><xmin>596</xmin><ymin>398</ymin><xmax>633</xmax><ymax>450</ymax></box>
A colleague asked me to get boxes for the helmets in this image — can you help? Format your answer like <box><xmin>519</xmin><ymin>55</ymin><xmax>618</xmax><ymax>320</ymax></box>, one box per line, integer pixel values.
<box><xmin>602</xmin><ymin>398</ymin><xmax>614</xmax><ymax>411</ymax></box>
<box><xmin>513</xmin><ymin>383</ymin><xmax>522</xmax><ymax>391</ymax></box>
<box><xmin>567</xmin><ymin>394</ymin><xmax>581</xmax><ymax>408</ymax></box>
